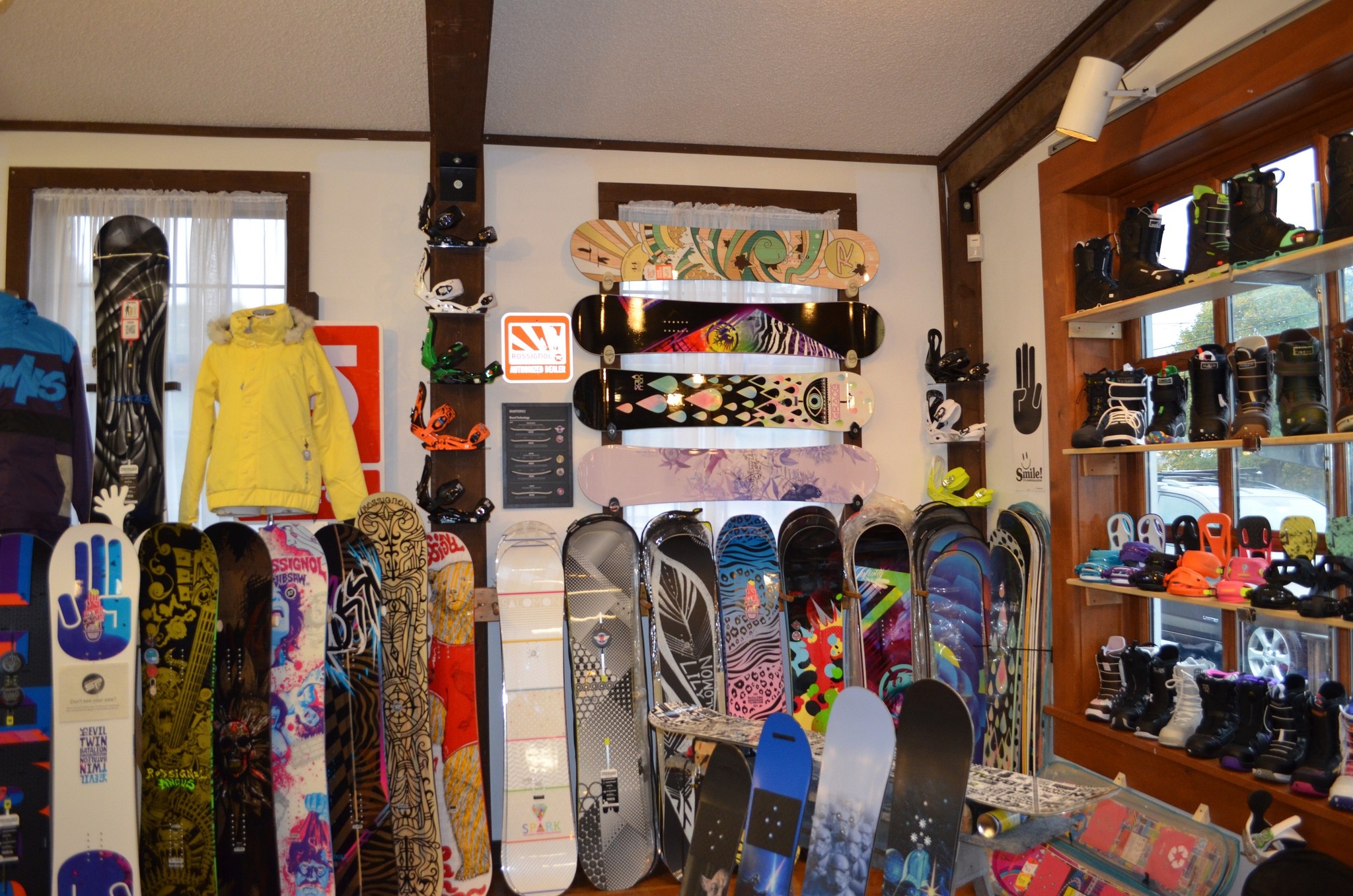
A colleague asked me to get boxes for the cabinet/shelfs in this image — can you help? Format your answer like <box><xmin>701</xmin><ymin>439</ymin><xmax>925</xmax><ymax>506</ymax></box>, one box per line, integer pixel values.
<box><xmin>1036</xmin><ymin>2</ymin><xmax>1353</xmax><ymax>865</ymax></box>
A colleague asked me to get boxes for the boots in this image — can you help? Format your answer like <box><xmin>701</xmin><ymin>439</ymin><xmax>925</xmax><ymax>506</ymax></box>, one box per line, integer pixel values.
<box><xmin>1073</xmin><ymin>131</ymin><xmax>1352</xmax><ymax>810</ymax></box>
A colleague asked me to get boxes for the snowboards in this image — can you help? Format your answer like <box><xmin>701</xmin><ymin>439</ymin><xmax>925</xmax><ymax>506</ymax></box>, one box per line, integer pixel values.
<box><xmin>312</xmin><ymin>523</ymin><xmax>400</xmax><ymax>896</ymax></box>
<box><xmin>564</xmin><ymin>513</ymin><xmax>658</xmax><ymax>893</ymax></box>
<box><xmin>800</xmin><ymin>685</ymin><xmax>897</xmax><ymax>895</ymax></box>
<box><xmin>134</xmin><ymin>521</ymin><xmax>218</xmax><ymax>896</ymax></box>
<box><xmin>648</xmin><ymin>697</ymin><xmax>1122</xmax><ymax>816</ymax></box>
<box><xmin>83</xmin><ymin>211</ymin><xmax>173</xmax><ymax>538</ymax></box>
<box><xmin>256</xmin><ymin>521</ymin><xmax>337</xmax><ymax>895</ymax></box>
<box><xmin>736</xmin><ymin>708</ymin><xmax>812</xmax><ymax>896</ymax></box>
<box><xmin>569</xmin><ymin>220</ymin><xmax>881</xmax><ymax>289</ymax></box>
<box><xmin>577</xmin><ymin>444</ymin><xmax>877</xmax><ymax>509</ymax></box>
<box><xmin>638</xmin><ymin>511</ymin><xmax>726</xmax><ymax>879</ymax></box>
<box><xmin>879</xmin><ymin>679</ymin><xmax>972</xmax><ymax>894</ymax></box>
<box><xmin>680</xmin><ymin>741</ymin><xmax>754</xmax><ymax>896</ymax></box>
<box><xmin>43</xmin><ymin>522</ymin><xmax>141</xmax><ymax>896</ymax></box>
<box><xmin>197</xmin><ymin>522</ymin><xmax>281</xmax><ymax>896</ymax></box>
<box><xmin>712</xmin><ymin>515</ymin><xmax>786</xmax><ymax>729</ymax></box>
<box><xmin>775</xmin><ymin>505</ymin><xmax>848</xmax><ymax>738</ymax></box>
<box><xmin>841</xmin><ymin>493</ymin><xmax>1055</xmax><ymax>780</ymax></box>
<box><xmin>494</xmin><ymin>520</ymin><xmax>578</xmax><ymax>896</ymax></box>
<box><xmin>572</xmin><ymin>294</ymin><xmax>885</xmax><ymax>364</ymax></box>
<box><xmin>419</xmin><ymin>531</ymin><xmax>493</xmax><ymax>892</ymax></box>
<box><xmin>573</xmin><ymin>369</ymin><xmax>876</xmax><ymax>433</ymax></box>
<box><xmin>353</xmin><ymin>492</ymin><xmax>444</xmax><ymax>896</ymax></box>
